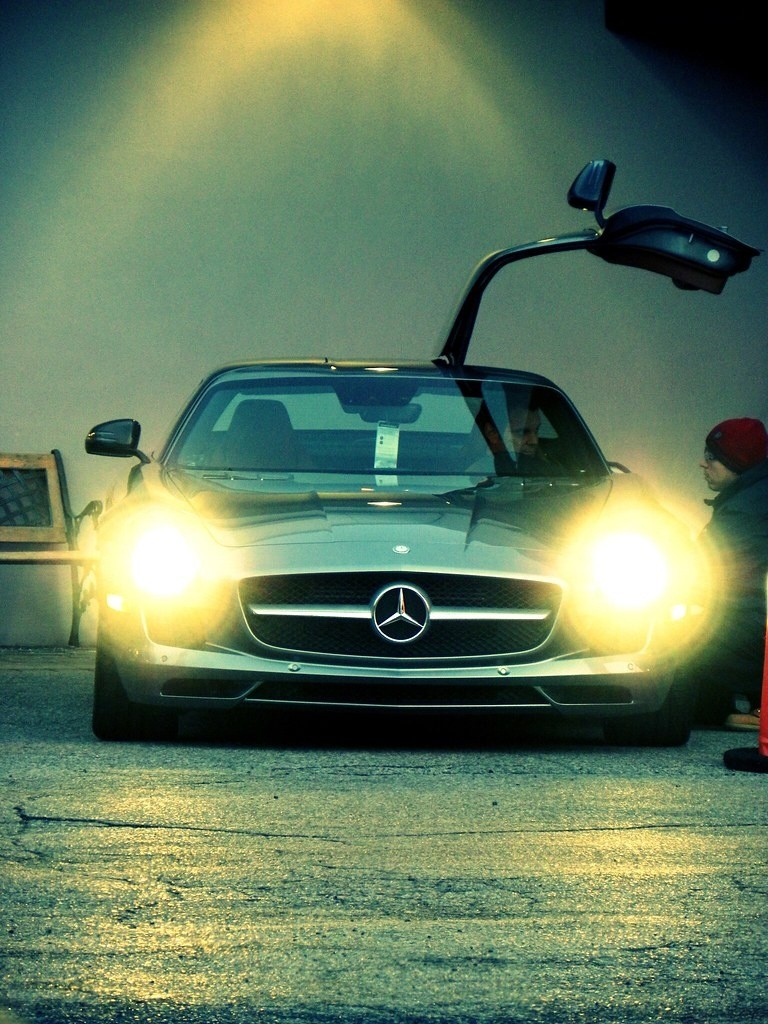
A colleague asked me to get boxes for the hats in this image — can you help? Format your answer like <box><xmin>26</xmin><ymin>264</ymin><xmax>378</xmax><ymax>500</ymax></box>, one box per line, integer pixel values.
<box><xmin>706</xmin><ymin>418</ymin><xmax>768</xmax><ymax>475</ymax></box>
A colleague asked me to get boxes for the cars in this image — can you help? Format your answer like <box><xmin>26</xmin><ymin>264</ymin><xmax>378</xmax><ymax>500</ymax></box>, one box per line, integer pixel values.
<box><xmin>86</xmin><ymin>158</ymin><xmax>763</xmax><ymax>749</ymax></box>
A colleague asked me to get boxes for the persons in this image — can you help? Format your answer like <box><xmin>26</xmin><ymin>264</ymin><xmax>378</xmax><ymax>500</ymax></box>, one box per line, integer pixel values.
<box><xmin>687</xmin><ymin>417</ymin><xmax>768</xmax><ymax>729</ymax></box>
<box><xmin>449</xmin><ymin>393</ymin><xmax>570</xmax><ymax>481</ymax></box>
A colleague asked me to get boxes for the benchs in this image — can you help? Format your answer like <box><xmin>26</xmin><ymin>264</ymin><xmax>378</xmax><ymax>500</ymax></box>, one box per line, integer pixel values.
<box><xmin>0</xmin><ymin>448</ymin><xmax>104</xmax><ymax>648</ymax></box>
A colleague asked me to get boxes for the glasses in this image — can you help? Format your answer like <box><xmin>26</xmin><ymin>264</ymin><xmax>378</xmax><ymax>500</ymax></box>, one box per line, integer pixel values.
<box><xmin>499</xmin><ymin>424</ymin><xmax>541</xmax><ymax>436</ymax></box>
<box><xmin>704</xmin><ymin>448</ymin><xmax>714</xmax><ymax>461</ymax></box>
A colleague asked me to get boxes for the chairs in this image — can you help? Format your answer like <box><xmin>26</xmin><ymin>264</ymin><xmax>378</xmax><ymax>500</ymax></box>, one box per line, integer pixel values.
<box><xmin>222</xmin><ymin>399</ymin><xmax>300</xmax><ymax>469</ymax></box>
